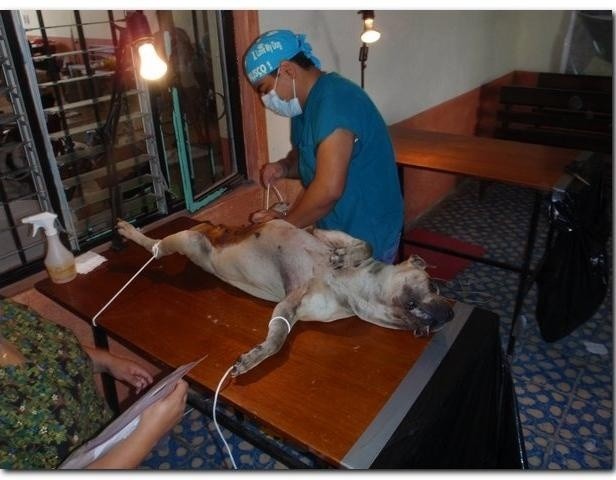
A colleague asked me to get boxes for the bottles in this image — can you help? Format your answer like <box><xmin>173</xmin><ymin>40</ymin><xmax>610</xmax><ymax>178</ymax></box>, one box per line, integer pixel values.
<box><xmin>243</xmin><ymin>29</ymin><xmax>320</xmax><ymax>85</ymax></box>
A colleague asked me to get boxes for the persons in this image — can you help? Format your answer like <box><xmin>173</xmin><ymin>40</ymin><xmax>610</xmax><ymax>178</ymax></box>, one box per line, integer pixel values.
<box><xmin>0</xmin><ymin>293</ymin><xmax>189</xmax><ymax>470</ymax></box>
<box><xmin>242</xmin><ymin>28</ymin><xmax>405</xmax><ymax>267</ymax></box>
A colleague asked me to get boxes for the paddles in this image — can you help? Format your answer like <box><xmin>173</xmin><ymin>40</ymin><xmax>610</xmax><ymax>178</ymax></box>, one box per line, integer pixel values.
<box><xmin>391</xmin><ymin>226</ymin><xmax>485</xmax><ymax>280</ymax></box>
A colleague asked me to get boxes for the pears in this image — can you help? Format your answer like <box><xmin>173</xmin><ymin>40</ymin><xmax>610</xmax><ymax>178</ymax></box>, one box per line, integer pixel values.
<box><xmin>20</xmin><ymin>210</ymin><xmax>78</xmax><ymax>285</ymax></box>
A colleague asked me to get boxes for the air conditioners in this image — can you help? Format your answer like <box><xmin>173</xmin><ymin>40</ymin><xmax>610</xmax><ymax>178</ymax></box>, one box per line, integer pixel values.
<box><xmin>114</xmin><ymin>200</ymin><xmax>459</xmax><ymax>380</ymax></box>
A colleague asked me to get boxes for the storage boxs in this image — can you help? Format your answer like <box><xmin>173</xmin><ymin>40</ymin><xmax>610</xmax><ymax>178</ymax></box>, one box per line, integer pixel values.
<box><xmin>493</xmin><ymin>86</ymin><xmax>611</xmax><ymax>160</ymax></box>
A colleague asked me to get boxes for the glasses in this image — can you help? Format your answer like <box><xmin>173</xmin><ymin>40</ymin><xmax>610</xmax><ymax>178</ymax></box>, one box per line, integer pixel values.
<box><xmin>79</xmin><ymin>11</ymin><xmax>167</xmax><ymax>247</ymax></box>
<box><xmin>352</xmin><ymin>10</ymin><xmax>380</xmax><ymax>92</ymax></box>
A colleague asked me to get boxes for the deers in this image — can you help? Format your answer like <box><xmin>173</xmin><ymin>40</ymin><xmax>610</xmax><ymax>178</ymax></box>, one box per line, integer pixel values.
<box><xmin>259</xmin><ymin>61</ymin><xmax>306</xmax><ymax>121</ymax></box>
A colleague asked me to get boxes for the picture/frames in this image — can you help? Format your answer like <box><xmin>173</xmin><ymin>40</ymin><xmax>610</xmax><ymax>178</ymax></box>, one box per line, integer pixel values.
<box><xmin>390</xmin><ymin>125</ymin><xmax>584</xmax><ymax>359</ymax></box>
<box><xmin>31</xmin><ymin>216</ymin><xmax>500</xmax><ymax>471</ymax></box>
